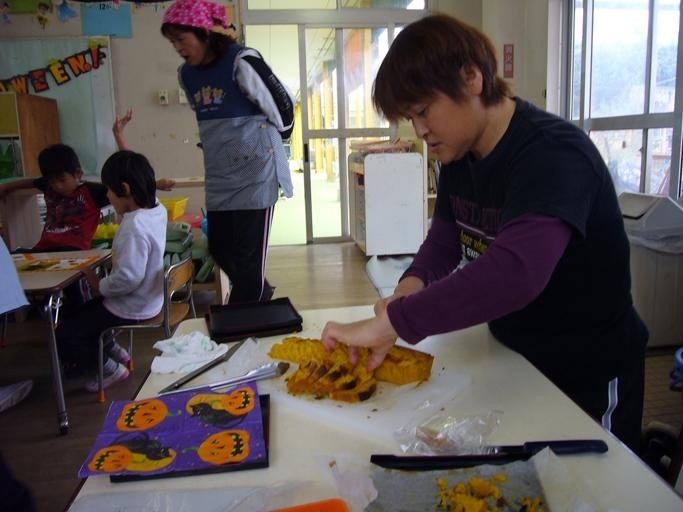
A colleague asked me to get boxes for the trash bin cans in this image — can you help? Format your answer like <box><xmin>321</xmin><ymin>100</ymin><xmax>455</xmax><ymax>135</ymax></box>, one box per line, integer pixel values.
<box><xmin>616</xmin><ymin>191</ymin><xmax>683</xmax><ymax>349</ymax></box>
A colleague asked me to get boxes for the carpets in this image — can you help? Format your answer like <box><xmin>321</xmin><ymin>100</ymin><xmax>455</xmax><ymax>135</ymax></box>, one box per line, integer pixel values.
<box><xmin>364</xmin><ymin>255</ymin><xmax>414</xmax><ymax>299</ymax></box>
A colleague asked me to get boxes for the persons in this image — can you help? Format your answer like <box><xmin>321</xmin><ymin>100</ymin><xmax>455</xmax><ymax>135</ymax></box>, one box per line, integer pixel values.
<box><xmin>161</xmin><ymin>0</ymin><xmax>294</xmax><ymax>304</ymax></box>
<box><xmin>1</xmin><ymin>146</ymin><xmax>174</xmax><ymax>255</ymax></box>
<box><xmin>321</xmin><ymin>15</ymin><xmax>650</xmax><ymax>451</ymax></box>
<box><xmin>89</xmin><ymin>109</ymin><xmax>168</xmax><ymax>390</ymax></box>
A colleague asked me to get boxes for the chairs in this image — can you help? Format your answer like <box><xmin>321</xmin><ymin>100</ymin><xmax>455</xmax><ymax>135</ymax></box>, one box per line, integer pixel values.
<box><xmin>98</xmin><ymin>251</ymin><xmax>198</xmax><ymax>403</ymax></box>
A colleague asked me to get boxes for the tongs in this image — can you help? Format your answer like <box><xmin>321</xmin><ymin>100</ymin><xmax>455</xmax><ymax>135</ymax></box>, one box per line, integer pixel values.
<box><xmin>157</xmin><ymin>338</ymin><xmax>290</xmax><ymax>397</ymax></box>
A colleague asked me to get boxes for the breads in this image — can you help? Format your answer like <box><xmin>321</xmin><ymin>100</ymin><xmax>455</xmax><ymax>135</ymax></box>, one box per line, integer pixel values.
<box><xmin>271</xmin><ymin>333</ymin><xmax>433</xmax><ymax>400</ymax></box>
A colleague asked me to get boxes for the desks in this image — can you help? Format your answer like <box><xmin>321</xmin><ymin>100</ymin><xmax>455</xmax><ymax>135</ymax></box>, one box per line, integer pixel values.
<box><xmin>64</xmin><ymin>305</ymin><xmax>683</xmax><ymax>512</ymax></box>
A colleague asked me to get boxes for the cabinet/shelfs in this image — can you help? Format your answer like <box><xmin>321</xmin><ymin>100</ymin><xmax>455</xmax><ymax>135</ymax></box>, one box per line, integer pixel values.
<box><xmin>397</xmin><ymin>117</ymin><xmax>443</xmax><ymax>239</ymax></box>
<box><xmin>348</xmin><ymin>151</ymin><xmax>424</xmax><ymax>257</ymax></box>
<box><xmin>0</xmin><ymin>92</ymin><xmax>61</xmax><ymax>191</ymax></box>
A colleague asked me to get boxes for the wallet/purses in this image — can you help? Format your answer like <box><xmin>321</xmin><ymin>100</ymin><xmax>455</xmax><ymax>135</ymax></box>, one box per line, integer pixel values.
<box><xmin>203</xmin><ymin>296</ymin><xmax>304</xmax><ymax>346</ymax></box>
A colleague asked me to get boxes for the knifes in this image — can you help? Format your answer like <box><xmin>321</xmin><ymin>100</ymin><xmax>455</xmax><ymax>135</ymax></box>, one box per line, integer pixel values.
<box><xmin>488</xmin><ymin>440</ymin><xmax>608</xmax><ymax>456</ymax></box>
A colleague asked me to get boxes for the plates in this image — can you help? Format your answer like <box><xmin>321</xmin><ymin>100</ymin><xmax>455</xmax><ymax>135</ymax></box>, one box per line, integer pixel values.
<box><xmin>204</xmin><ymin>296</ymin><xmax>305</xmax><ymax>344</ymax></box>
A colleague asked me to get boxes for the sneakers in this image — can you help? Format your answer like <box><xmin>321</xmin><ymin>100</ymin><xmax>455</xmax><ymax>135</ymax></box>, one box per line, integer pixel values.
<box><xmin>0</xmin><ymin>379</ymin><xmax>34</xmax><ymax>413</ymax></box>
<box><xmin>82</xmin><ymin>362</ymin><xmax>131</xmax><ymax>393</ymax></box>
<box><xmin>112</xmin><ymin>347</ymin><xmax>133</xmax><ymax>364</ymax></box>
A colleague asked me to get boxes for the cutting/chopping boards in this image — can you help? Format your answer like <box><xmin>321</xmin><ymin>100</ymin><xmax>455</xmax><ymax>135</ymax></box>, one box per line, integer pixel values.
<box><xmin>258</xmin><ymin>362</ymin><xmax>470</xmax><ymax>440</ymax></box>
